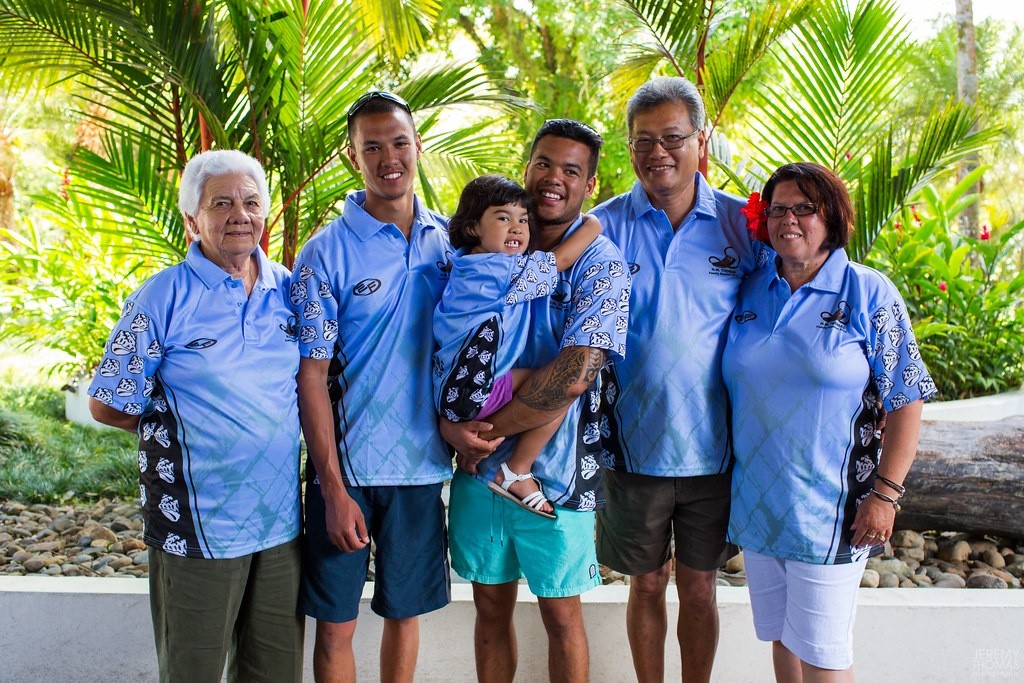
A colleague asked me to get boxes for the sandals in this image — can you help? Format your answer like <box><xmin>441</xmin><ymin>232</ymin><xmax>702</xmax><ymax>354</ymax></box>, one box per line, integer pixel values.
<box><xmin>487</xmin><ymin>462</ymin><xmax>558</xmax><ymax>519</ymax></box>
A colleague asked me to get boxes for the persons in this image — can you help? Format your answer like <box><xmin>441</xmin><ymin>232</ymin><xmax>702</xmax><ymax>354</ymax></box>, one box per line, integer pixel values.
<box><xmin>289</xmin><ymin>91</ymin><xmax>457</xmax><ymax>683</ymax></box>
<box><xmin>439</xmin><ymin>121</ymin><xmax>633</xmax><ymax>683</ymax></box>
<box><xmin>430</xmin><ymin>175</ymin><xmax>603</xmax><ymax>519</ymax></box>
<box><xmin>88</xmin><ymin>149</ymin><xmax>304</xmax><ymax>683</ymax></box>
<box><xmin>583</xmin><ymin>75</ymin><xmax>887</xmax><ymax>683</ymax></box>
<box><xmin>723</xmin><ymin>163</ymin><xmax>937</xmax><ymax>683</ymax></box>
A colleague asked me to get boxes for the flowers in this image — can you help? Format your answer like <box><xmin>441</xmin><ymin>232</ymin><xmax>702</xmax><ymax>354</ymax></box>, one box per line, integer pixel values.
<box><xmin>739</xmin><ymin>191</ymin><xmax>772</xmax><ymax>240</ymax></box>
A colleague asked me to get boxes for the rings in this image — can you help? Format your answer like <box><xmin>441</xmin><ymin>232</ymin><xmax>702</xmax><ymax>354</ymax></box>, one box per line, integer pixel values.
<box><xmin>867</xmin><ymin>533</ymin><xmax>876</xmax><ymax>538</ymax></box>
<box><xmin>876</xmin><ymin>535</ymin><xmax>885</xmax><ymax>542</ymax></box>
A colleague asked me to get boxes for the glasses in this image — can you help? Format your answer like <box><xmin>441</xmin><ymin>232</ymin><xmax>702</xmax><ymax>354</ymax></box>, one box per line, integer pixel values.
<box><xmin>763</xmin><ymin>202</ymin><xmax>820</xmax><ymax>218</ymax></box>
<box><xmin>347</xmin><ymin>93</ymin><xmax>411</xmax><ymax>135</ymax></box>
<box><xmin>631</xmin><ymin>129</ymin><xmax>703</xmax><ymax>152</ymax></box>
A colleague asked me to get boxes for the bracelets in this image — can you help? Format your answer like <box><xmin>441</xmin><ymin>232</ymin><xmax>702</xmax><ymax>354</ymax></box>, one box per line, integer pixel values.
<box><xmin>870</xmin><ymin>474</ymin><xmax>905</xmax><ymax>512</ymax></box>
